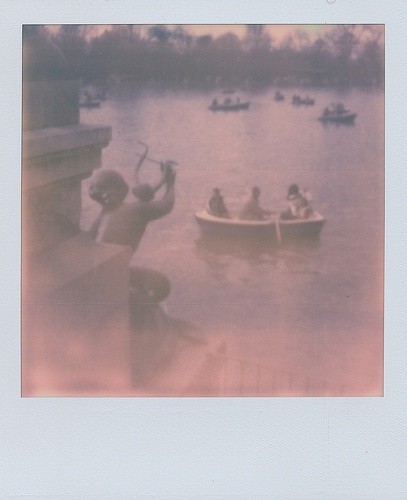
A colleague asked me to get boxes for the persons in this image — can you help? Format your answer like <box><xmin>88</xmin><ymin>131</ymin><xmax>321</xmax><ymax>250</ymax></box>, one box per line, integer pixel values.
<box><xmin>74</xmin><ymin>87</ymin><xmax>356</xmax><ymax>226</ymax></box>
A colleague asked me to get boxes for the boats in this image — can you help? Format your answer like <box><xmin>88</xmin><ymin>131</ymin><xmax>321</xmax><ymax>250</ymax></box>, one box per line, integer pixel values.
<box><xmin>195</xmin><ymin>208</ymin><xmax>324</xmax><ymax>241</ymax></box>
<box><xmin>81</xmin><ymin>101</ymin><xmax>99</xmax><ymax>107</ymax></box>
<box><xmin>317</xmin><ymin>113</ymin><xmax>357</xmax><ymax>123</ymax></box>
<box><xmin>293</xmin><ymin>99</ymin><xmax>317</xmax><ymax>105</ymax></box>
<box><xmin>209</xmin><ymin>101</ymin><xmax>248</xmax><ymax>109</ymax></box>
